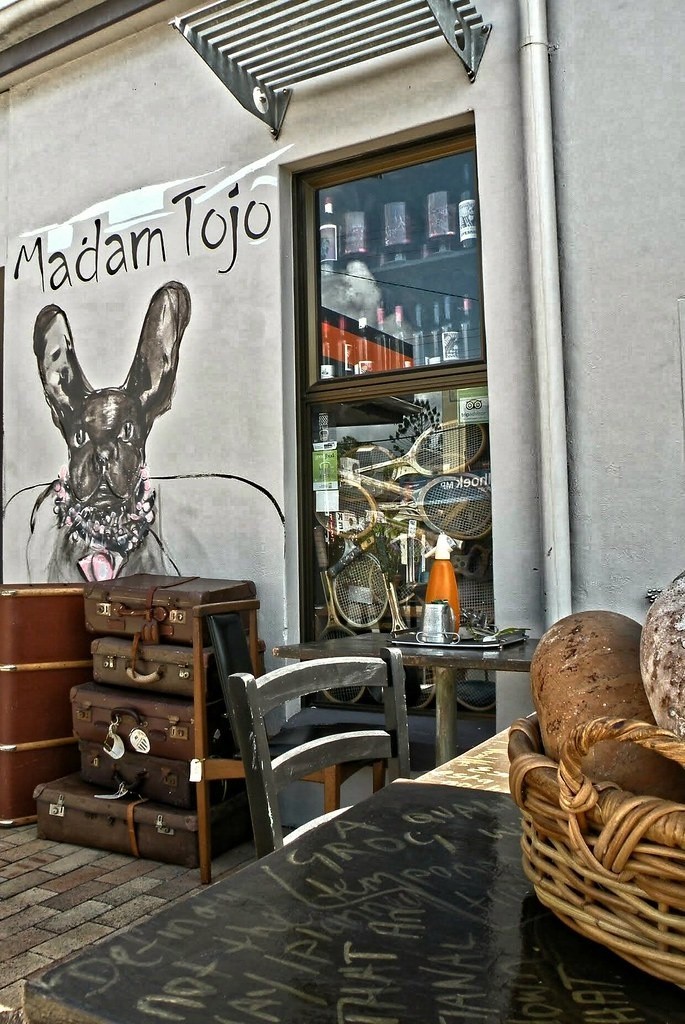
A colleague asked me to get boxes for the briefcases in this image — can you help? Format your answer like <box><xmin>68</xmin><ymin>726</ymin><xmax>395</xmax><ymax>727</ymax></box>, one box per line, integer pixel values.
<box><xmin>82</xmin><ymin>572</ymin><xmax>260</xmax><ymax>648</ymax></box>
<box><xmin>78</xmin><ymin>740</ymin><xmax>247</xmax><ymax>811</ymax></box>
<box><xmin>90</xmin><ymin>635</ymin><xmax>266</xmax><ymax>699</ymax></box>
<box><xmin>32</xmin><ymin>772</ymin><xmax>254</xmax><ymax>869</ymax></box>
<box><xmin>69</xmin><ymin>681</ymin><xmax>242</xmax><ymax>762</ymax></box>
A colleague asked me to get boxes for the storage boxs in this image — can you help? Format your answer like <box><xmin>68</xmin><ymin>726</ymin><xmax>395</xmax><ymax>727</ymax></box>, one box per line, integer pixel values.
<box><xmin>33</xmin><ymin>773</ymin><xmax>253</xmax><ymax>869</ymax></box>
<box><xmin>82</xmin><ymin>573</ymin><xmax>257</xmax><ymax>647</ymax></box>
<box><xmin>77</xmin><ymin>739</ymin><xmax>246</xmax><ymax>811</ymax></box>
<box><xmin>70</xmin><ymin>680</ymin><xmax>239</xmax><ymax>763</ymax></box>
<box><xmin>91</xmin><ymin>635</ymin><xmax>267</xmax><ymax>699</ymax></box>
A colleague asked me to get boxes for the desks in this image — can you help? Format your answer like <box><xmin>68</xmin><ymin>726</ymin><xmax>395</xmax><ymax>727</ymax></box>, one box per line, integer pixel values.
<box><xmin>273</xmin><ymin>632</ymin><xmax>542</xmax><ymax>767</ymax></box>
<box><xmin>0</xmin><ymin>711</ymin><xmax>685</xmax><ymax>1024</ymax></box>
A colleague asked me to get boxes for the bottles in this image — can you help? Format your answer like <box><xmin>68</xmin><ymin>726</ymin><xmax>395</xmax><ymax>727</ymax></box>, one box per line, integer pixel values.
<box><xmin>425</xmin><ymin>174</ymin><xmax>457</xmax><ymax>240</ymax></box>
<box><xmin>441</xmin><ymin>297</ymin><xmax>462</xmax><ymax>362</ymax></box>
<box><xmin>354</xmin><ymin>340</ymin><xmax>359</xmax><ymax>375</ymax></box>
<box><xmin>459</xmin><ymin>164</ymin><xmax>477</xmax><ymax>248</ymax></box>
<box><xmin>411</xmin><ymin>304</ymin><xmax>425</xmax><ymax>367</ymax></box>
<box><xmin>422</xmin><ymin>534</ymin><xmax>460</xmax><ymax>638</ymax></box>
<box><xmin>320</xmin><ymin>317</ymin><xmax>335</xmax><ymax>379</ymax></box>
<box><xmin>358</xmin><ymin>312</ymin><xmax>374</xmax><ymax>374</ymax></box>
<box><xmin>472</xmin><ymin>299</ymin><xmax>481</xmax><ymax>360</ymax></box>
<box><xmin>344</xmin><ymin>190</ymin><xmax>369</xmax><ymax>258</ymax></box>
<box><xmin>320</xmin><ymin>195</ymin><xmax>343</xmax><ymax>271</ymax></box>
<box><xmin>338</xmin><ymin>316</ymin><xmax>354</xmax><ymax>376</ymax></box>
<box><xmin>375</xmin><ymin>308</ymin><xmax>391</xmax><ymax>372</ymax></box>
<box><xmin>390</xmin><ymin>306</ymin><xmax>404</xmax><ymax>369</ymax></box>
<box><xmin>429</xmin><ymin>302</ymin><xmax>443</xmax><ymax>364</ymax></box>
<box><xmin>381</xmin><ymin>181</ymin><xmax>412</xmax><ymax>254</ymax></box>
<box><xmin>461</xmin><ymin>294</ymin><xmax>472</xmax><ymax>360</ymax></box>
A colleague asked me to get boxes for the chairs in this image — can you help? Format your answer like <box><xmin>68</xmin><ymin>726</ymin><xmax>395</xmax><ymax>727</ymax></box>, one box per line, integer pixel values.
<box><xmin>226</xmin><ymin>647</ymin><xmax>411</xmax><ymax>859</ymax></box>
<box><xmin>191</xmin><ymin>599</ymin><xmax>388</xmax><ymax>885</ymax></box>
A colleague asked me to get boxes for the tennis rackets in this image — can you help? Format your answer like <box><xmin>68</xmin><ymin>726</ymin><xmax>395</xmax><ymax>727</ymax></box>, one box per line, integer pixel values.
<box><xmin>353</xmin><ymin>442</ymin><xmax>486</xmax><ymax>481</ymax></box>
<box><xmin>355</xmin><ymin>416</ymin><xmax>484</xmax><ymax>478</ymax></box>
<box><xmin>313</xmin><ymin>480</ymin><xmax>465</xmax><ymax>555</ymax></box>
<box><xmin>367</xmin><ymin>533</ymin><xmax>426</xmax><ymax>604</ymax></box>
<box><xmin>336</xmin><ymin>444</ymin><xmax>471</xmax><ymax>503</ymax></box>
<box><xmin>343</xmin><ymin>471</ymin><xmax>492</xmax><ymax>540</ymax></box>
<box><xmin>332</xmin><ymin>531</ymin><xmax>388</xmax><ymax>629</ymax></box>
<box><xmin>313</xmin><ymin>524</ymin><xmax>358</xmax><ymax>640</ymax></box>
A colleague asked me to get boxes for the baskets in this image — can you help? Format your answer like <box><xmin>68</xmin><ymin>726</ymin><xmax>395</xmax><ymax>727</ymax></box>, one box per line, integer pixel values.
<box><xmin>507</xmin><ymin>711</ymin><xmax>685</xmax><ymax>991</ymax></box>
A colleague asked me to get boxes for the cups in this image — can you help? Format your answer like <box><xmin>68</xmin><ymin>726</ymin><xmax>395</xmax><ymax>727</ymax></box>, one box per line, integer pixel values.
<box><xmin>421</xmin><ymin>603</ymin><xmax>454</xmax><ymax>643</ymax></box>
